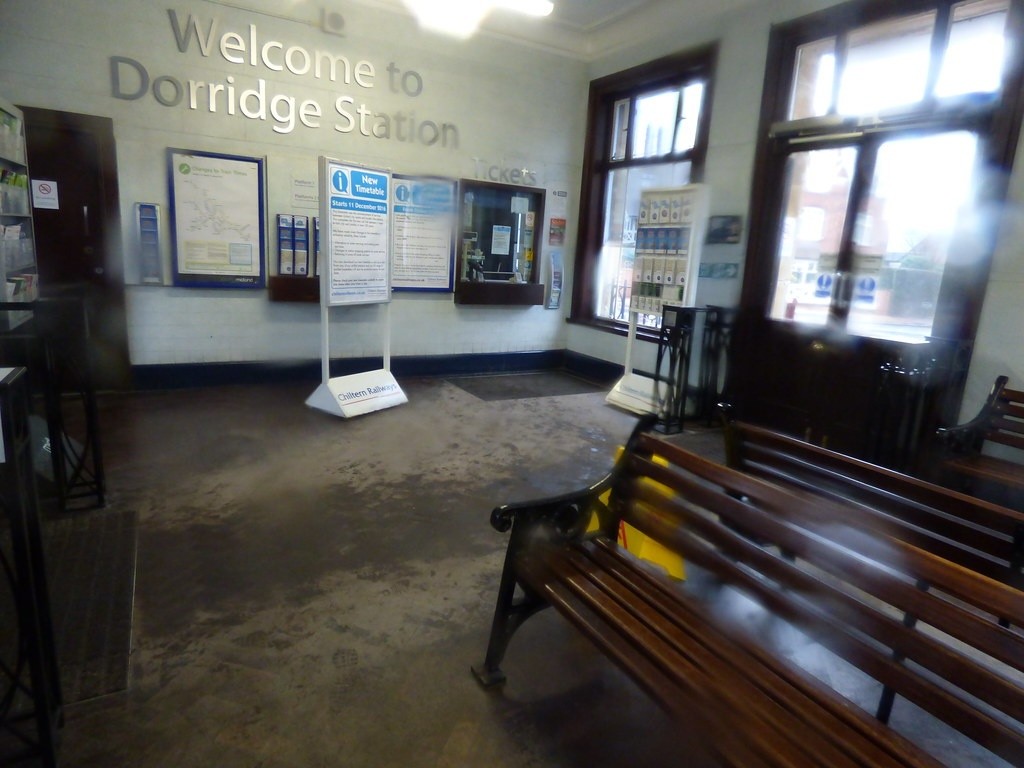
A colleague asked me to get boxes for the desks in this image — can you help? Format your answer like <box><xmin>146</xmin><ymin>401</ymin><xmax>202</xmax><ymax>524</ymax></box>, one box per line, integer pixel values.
<box><xmin>0</xmin><ymin>367</ymin><xmax>67</xmax><ymax>768</ymax></box>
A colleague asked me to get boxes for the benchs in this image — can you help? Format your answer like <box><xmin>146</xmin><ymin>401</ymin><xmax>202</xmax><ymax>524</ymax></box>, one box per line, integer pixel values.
<box><xmin>709</xmin><ymin>401</ymin><xmax>1024</xmax><ymax>630</ymax></box>
<box><xmin>470</xmin><ymin>414</ymin><xmax>1024</xmax><ymax>768</ymax></box>
<box><xmin>936</xmin><ymin>377</ymin><xmax>1024</xmax><ymax>538</ymax></box>
